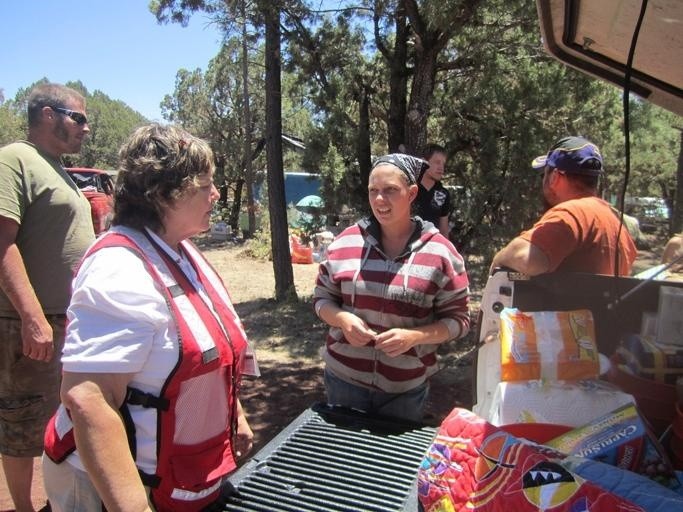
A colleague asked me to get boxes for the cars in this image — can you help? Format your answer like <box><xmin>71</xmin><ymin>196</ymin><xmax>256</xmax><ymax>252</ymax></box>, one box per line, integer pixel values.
<box><xmin>63</xmin><ymin>168</ymin><xmax>114</xmax><ymax>237</ymax></box>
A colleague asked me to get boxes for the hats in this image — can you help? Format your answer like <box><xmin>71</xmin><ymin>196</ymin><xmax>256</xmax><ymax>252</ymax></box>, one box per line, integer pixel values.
<box><xmin>530</xmin><ymin>136</ymin><xmax>604</xmax><ymax>178</ymax></box>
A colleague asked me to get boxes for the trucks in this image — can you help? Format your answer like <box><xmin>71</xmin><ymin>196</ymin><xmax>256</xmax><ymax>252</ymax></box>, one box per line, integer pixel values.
<box><xmin>208</xmin><ymin>1</ymin><xmax>683</xmax><ymax>509</ymax></box>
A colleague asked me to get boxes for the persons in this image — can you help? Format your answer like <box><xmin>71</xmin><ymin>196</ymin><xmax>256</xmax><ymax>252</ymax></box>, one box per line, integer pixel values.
<box><xmin>488</xmin><ymin>134</ymin><xmax>637</xmax><ymax>275</ymax></box>
<box><xmin>312</xmin><ymin>154</ymin><xmax>470</xmax><ymax>418</ymax></box>
<box><xmin>0</xmin><ymin>82</ymin><xmax>96</xmax><ymax>512</ymax></box>
<box><xmin>41</xmin><ymin>121</ymin><xmax>254</xmax><ymax>512</ymax></box>
<box><xmin>410</xmin><ymin>144</ymin><xmax>451</xmax><ymax>239</ymax></box>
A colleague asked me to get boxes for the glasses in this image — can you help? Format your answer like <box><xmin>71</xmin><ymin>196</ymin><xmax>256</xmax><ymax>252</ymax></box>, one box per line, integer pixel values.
<box><xmin>51</xmin><ymin>106</ymin><xmax>86</xmax><ymax>125</ymax></box>
